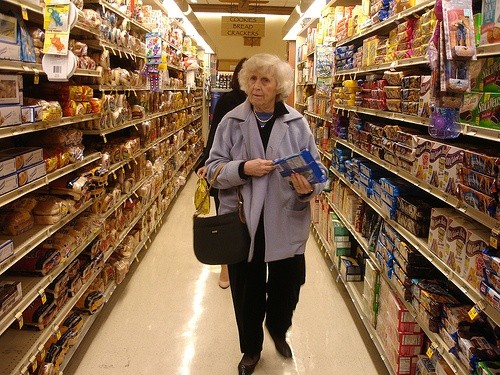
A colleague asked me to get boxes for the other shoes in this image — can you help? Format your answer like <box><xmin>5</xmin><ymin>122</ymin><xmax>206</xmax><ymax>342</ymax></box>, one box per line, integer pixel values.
<box><xmin>218</xmin><ymin>273</ymin><xmax>230</xmax><ymax>289</ymax></box>
<box><xmin>273</xmin><ymin>337</ymin><xmax>292</xmax><ymax>358</ymax></box>
<box><xmin>238</xmin><ymin>351</ymin><xmax>261</xmax><ymax>375</ymax></box>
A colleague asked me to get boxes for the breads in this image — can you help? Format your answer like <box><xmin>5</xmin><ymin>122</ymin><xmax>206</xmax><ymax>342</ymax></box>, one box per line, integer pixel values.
<box><xmin>0</xmin><ymin>28</ymin><xmax>148</xmax><ymax>375</ymax></box>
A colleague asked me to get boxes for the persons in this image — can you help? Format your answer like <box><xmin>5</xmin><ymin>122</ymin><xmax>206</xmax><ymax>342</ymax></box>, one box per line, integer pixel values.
<box><xmin>205</xmin><ymin>54</ymin><xmax>328</xmax><ymax>375</ymax></box>
<box><xmin>195</xmin><ymin>57</ymin><xmax>248</xmax><ymax>289</ymax></box>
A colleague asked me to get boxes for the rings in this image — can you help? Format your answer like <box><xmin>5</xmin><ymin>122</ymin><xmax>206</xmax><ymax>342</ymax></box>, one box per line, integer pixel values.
<box><xmin>296</xmin><ymin>186</ymin><xmax>300</xmax><ymax>188</ymax></box>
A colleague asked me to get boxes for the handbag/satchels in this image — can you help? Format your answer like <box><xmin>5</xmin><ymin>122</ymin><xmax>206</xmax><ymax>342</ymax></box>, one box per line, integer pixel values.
<box><xmin>193</xmin><ymin>164</ymin><xmax>251</xmax><ymax>265</ymax></box>
<box><xmin>194</xmin><ymin>176</ymin><xmax>210</xmax><ymax>215</ymax></box>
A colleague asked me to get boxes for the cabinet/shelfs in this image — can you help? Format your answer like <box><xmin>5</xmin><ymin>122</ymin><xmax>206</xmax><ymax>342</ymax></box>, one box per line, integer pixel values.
<box><xmin>294</xmin><ymin>0</ymin><xmax>500</xmax><ymax>375</ymax></box>
<box><xmin>0</xmin><ymin>0</ymin><xmax>216</xmax><ymax>375</ymax></box>
